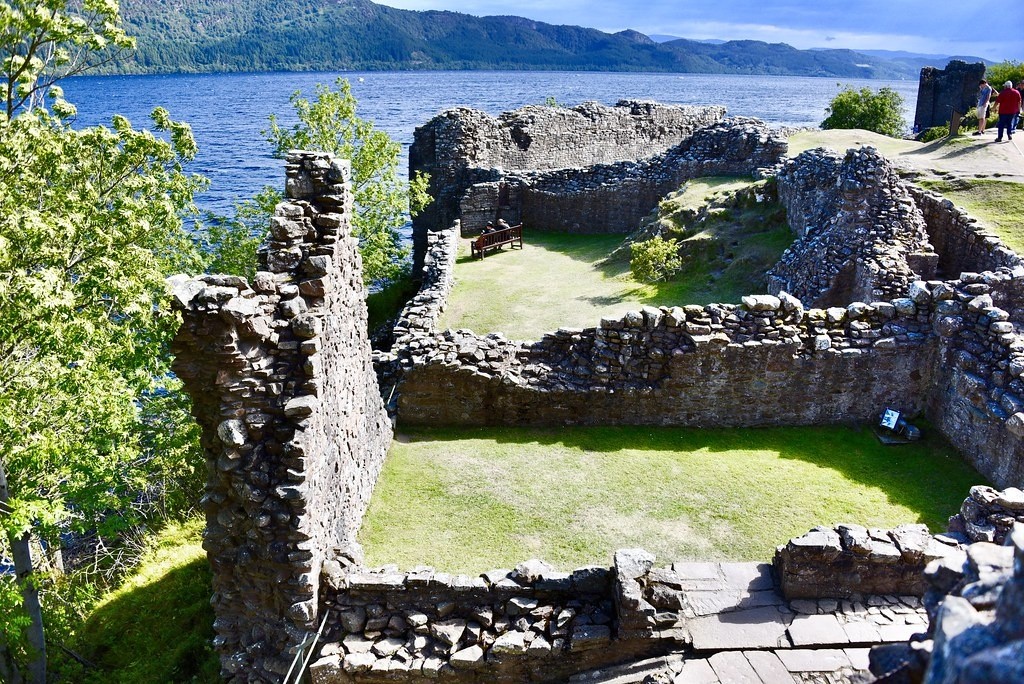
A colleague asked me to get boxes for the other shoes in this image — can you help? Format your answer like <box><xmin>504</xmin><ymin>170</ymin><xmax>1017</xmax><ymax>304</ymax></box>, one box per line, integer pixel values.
<box><xmin>1008</xmin><ymin>134</ymin><xmax>1012</xmax><ymax>139</ymax></box>
<box><xmin>972</xmin><ymin>130</ymin><xmax>985</xmax><ymax>136</ymax></box>
<box><xmin>995</xmin><ymin>138</ymin><xmax>1001</xmax><ymax>142</ymax></box>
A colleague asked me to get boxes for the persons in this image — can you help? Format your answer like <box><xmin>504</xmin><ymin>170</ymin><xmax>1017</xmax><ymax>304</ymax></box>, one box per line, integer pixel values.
<box><xmin>972</xmin><ymin>79</ymin><xmax>1024</xmax><ymax>142</ymax></box>
<box><xmin>493</xmin><ymin>219</ymin><xmax>511</xmax><ymax>250</ymax></box>
<box><xmin>477</xmin><ymin>221</ymin><xmax>496</xmax><ymax>253</ymax></box>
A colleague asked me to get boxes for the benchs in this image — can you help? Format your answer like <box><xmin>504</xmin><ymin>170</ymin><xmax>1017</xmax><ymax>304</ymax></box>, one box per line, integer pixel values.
<box><xmin>471</xmin><ymin>222</ymin><xmax>523</xmax><ymax>260</ymax></box>
